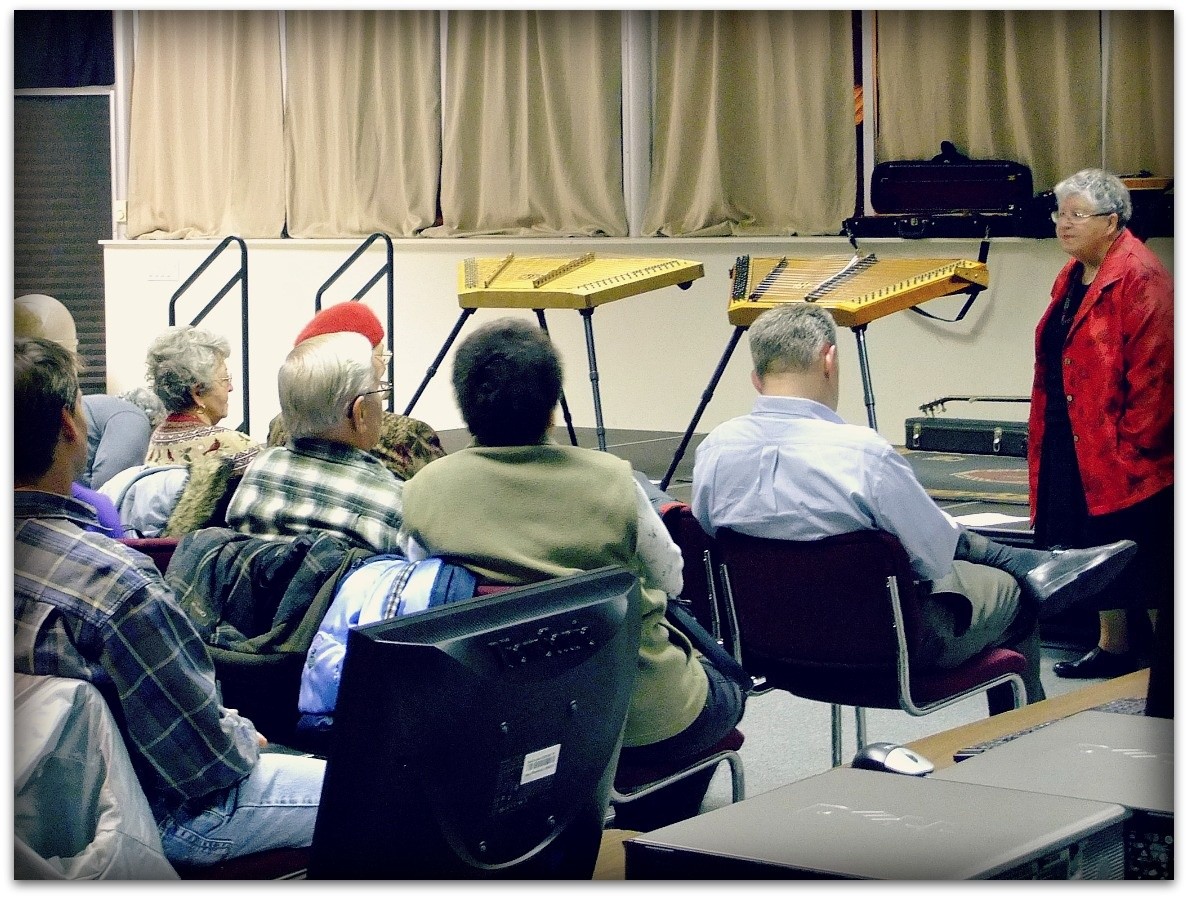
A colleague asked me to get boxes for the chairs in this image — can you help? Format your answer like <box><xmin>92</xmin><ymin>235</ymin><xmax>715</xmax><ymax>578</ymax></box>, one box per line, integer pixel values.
<box><xmin>475</xmin><ymin>501</ymin><xmax>1027</xmax><ymax>805</ymax></box>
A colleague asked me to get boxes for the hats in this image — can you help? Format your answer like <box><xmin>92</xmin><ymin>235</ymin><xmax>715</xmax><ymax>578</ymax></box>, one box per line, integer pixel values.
<box><xmin>294</xmin><ymin>302</ymin><xmax>383</xmax><ymax>348</ymax></box>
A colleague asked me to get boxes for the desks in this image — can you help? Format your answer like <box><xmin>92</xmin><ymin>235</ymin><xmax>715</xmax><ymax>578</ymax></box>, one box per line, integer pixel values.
<box><xmin>902</xmin><ymin>667</ymin><xmax>1151</xmax><ymax>770</ymax></box>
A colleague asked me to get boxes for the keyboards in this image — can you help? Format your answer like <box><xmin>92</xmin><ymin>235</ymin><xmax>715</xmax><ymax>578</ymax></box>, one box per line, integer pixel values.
<box><xmin>954</xmin><ymin>698</ymin><xmax>1149</xmax><ymax>761</ymax></box>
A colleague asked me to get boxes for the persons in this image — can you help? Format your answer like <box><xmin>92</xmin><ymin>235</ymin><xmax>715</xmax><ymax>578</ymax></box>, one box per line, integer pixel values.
<box><xmin>75</xmin><ymin>388</ymin><xmax>168</xmax><ymax>492</ymax></box>
<box><xmin>144</xmin><ymin>328</ymin><xmax>258</xmax><ymax>538</ymax></box>
<box><xmin>14</xmin><ymin>336</ymin><xmax>327</xmax><ymax>864</ymax></box>
<box><xmin>225</xmin><ymin>332</ymin><xmax>404</xmax><ymax>555</ymax></box>
<box><xmin>692</xmin><ymin>304</ymin><xmax>1137</xmax><ymax>717</ymax></box>
<box><xmin>396</xmin><ymin>318</ymin><xmax>741</xmax><ymax>833</ymax></box>
<box><xmin>1027</xmin><ymin>168</ymin><xmax>1174</xmax><ymax>679</ymax></box>
<box><xmin>267</xmin><ymin>302</ymin><xmax>448</xmax><ymax>482</ymax></box>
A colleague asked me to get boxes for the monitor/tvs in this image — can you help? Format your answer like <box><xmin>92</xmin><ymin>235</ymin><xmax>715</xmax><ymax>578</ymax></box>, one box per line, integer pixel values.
<box><xmin>307</xmin><ymin>563</ymin><xmax>643</xmax><ymax>881</ymax></box>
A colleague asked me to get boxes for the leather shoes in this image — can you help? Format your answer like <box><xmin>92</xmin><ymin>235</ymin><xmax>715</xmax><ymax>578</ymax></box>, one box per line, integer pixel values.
<box><xmin>1027</xmin><ymin>540</ymin><xmax>1136</xmax><ymax>609</ymax></box>
<box><xmin>1053</xmin><ymin>646</ymin><xmax>1140</xmax><ymax>678</ymax></box>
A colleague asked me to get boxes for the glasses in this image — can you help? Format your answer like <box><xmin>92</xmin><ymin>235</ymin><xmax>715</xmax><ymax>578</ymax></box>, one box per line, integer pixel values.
<box><xmin>347</xmin><ymin>380</ymin><xmax>393</xmax><ymax>418</ymax></box>
<box><xmin>374</xmin><ymin>350</ymin><xmax>393</xmax><ymax>366</ymax></box>
<box><xmin>1052</xmin><ymin>209</ymin><xmax>1114</xmax><ymax>222</ymax></box>
<box><xmin>212</xmin><ymin>373</ymin><xmax>233</xmax><ymax>383</ymax></box>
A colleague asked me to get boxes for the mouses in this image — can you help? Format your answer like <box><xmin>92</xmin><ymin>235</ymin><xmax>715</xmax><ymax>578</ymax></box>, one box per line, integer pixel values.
<box><xmin>852</xmin><ymin>743</ymin><xmax>935</xmax><ymax>778</ymax></box>
